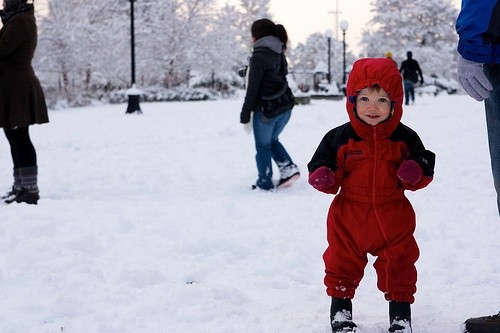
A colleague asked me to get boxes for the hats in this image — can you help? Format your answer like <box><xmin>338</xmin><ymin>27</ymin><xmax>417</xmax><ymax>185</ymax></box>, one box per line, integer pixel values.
<box><xmin>387</xmin><ymin>52</ymin><xmax>392</xmax><ymax>57</ymax></box>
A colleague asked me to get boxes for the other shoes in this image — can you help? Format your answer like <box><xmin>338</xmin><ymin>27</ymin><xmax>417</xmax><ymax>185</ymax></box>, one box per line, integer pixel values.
<box><xmin>388</xmin><ymin>302</ymin><xmax>411</xmax><ymax>333</ymax></box>
<box><xmin>6</xmin><ymin>189</ymin><xmax>39</xmax><ymax>204</ymax></box>
<box><xmin>2</xmin><ymin>185</ymin><xmax>17</xmax><ymax>199</ymax></box>
<box><xmin>253</xmin><ymin>185</ymin><xmax>275</xmax><ymax>190</ymax></box>
<box><xmin>277</xmin><ymin>170</ymin><xmax>300</xmax><ymax>188</ymax></box>
<box><xmin>465</xmin><ymin>311</ymin><xmax>500</xmax><ymax>333</ymax></box>
<box><xmin>329</xmin><ymin>298</ymin><xmax>356</xmax><ymax>333</ymax></box>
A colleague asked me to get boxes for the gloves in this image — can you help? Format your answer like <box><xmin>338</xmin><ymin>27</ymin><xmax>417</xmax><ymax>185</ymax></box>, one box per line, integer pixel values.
<box><xmin>457</xmin><ymin>58</ymin><xmax>493</xmax><ymax>102</ymax></box>
<box><xmin>420</xmin><ymin>80</ymin><xmax>423</xmax><ymax>84</ymax></box>
<box><xmin>307</xmin><ymin>167</ymin><xmax>335</xmax><ymax>188</ymax></box>
<box><xmin>397</xmin><ymin>160</ymin><xmax>422</xmax><ymax>185</ymax></box>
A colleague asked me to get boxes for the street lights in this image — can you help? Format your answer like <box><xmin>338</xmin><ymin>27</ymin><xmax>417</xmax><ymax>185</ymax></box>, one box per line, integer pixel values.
<box><xmin>340</xmin><ymin>19</ymin><xmax>348</xmax><ymax>95</ymax></box>
<box><xmin>326</xmin><ymin>31</ymin><xmax>335</xmax><ymax>87</ymax></box>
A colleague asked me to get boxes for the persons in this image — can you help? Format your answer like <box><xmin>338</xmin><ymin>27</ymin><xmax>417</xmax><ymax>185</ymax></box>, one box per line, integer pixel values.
<box><xmin>306</xmin><ymin>58</ymin><xmax>436</xmax><ymax>333</ymax></box>
<box><xmin>456</xmin><ymin>1</ymin><xmax>500</xmax><ymax>333</ymax></box>
<box><xmin>238</xmin><ymin>18</ymin><xmax>301</xmax><ymax>193</ymax></box>
<box><xmin>386</xmin><ymin>50</ymin><xmax>424</xmax><ymax>106</ymax></box>
<box><xmin>0</xmin><ymin>0</ymin><xmax>49</xmax><ymax>205</ymax></box>
